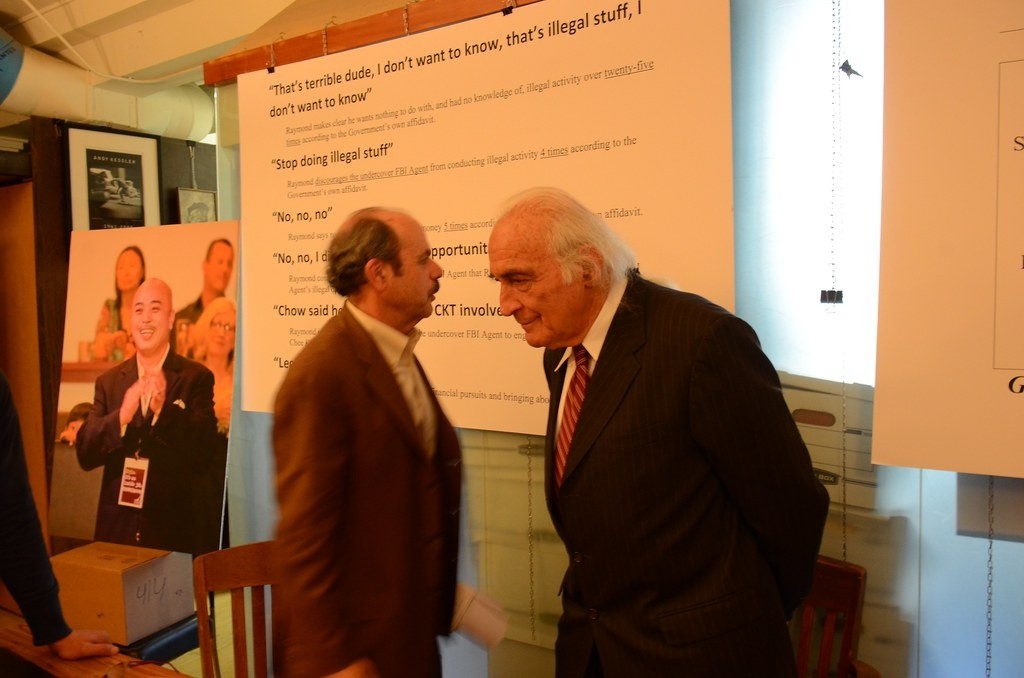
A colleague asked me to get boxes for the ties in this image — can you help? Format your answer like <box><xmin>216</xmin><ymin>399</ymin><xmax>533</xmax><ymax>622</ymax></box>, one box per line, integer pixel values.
<box><xmin>553</xmin><ymin>345</ymin><xmax>592</xmax><ymax>489</ymax></box>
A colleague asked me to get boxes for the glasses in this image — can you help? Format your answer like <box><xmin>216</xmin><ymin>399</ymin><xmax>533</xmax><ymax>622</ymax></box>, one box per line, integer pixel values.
<box><xmin>211</xmin><ymin>321</ymin><xmax>231</xmax><ymax>332</ymax></box>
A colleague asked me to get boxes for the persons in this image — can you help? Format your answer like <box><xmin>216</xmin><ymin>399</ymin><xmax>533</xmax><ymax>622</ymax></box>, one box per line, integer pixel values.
<box><xmin>0</xmin><ymin>377</ymin><xmax>118</xmax><ymax>661</ymax></box>
<box><xmin>266</xmin><ymin>207</ymin><xmax>466</xmax><ymax>678</ymax></box>
<box><xmin>75</xmin><ymin>237</ymin><xmax>239</xmax><ymax>551</ymax></box>
<box><xmin>487</xmin><ymin>187</ymin><xmax>831</xmax><ymax>678</ymax></box>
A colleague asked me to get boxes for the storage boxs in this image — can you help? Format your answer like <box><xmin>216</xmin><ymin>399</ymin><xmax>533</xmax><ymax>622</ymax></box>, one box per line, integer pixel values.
<box><xmin>48</xmin><ymin>541</ymin><xmax>193</xmax><ymax>648</ymax></box>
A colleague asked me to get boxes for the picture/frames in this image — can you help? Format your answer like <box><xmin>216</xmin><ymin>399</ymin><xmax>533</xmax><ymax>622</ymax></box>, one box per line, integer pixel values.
<box><xmin>177</xmin><ymin>187</ymin><xmax>218</xmax><ymax>223</ymax></box>
<box><xmin>63</xmin><ymin>120</ymin><xmax>165</xmax><ymax>262</ymax></box>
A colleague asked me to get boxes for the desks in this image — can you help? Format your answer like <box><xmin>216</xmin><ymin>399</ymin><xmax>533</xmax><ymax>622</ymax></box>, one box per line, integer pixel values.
<box><xmin>0</xmin><ymin>615</ymin><xmax>212</xmax><ymax>678</ymax></box>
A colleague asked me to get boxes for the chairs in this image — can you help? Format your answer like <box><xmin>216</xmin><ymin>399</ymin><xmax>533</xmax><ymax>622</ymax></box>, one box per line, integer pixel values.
<box><xmin>194</xmin><ymin>539</ymin><xmax>281</xmax><ymax>678</ymax></box>
<box><xmin>791</xmin><ymin>553</ymin><xmax>866</xmax><ymax>678</ymax></box>
